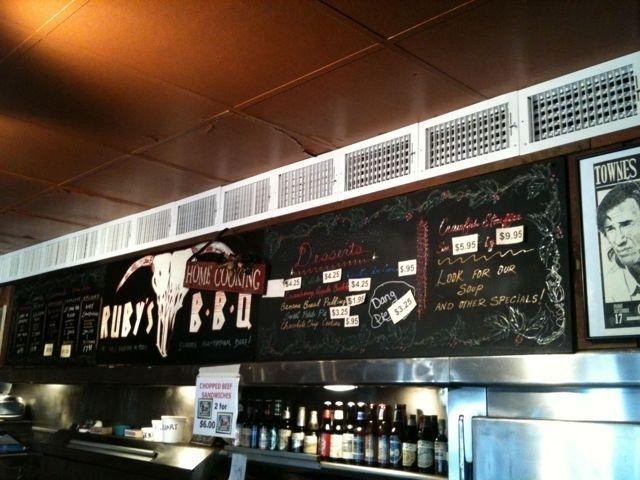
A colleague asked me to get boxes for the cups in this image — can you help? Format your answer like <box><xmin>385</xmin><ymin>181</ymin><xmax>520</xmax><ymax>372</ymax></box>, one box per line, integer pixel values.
<box><xmin>114</xmin><ymin>424</ymin><xmax>131</xmax><ymax>436</ymax></box>
<box><xmin>141</xmin><ymin>415</ymin><xmax>194</xmax><ymax>443</ymax></box>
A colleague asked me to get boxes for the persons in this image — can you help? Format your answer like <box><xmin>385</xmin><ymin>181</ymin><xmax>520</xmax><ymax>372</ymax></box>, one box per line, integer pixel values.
<box><xmin>596</xmin><ymin>182</ymin><xmax>640</xmax><ymax>302</ymax></box>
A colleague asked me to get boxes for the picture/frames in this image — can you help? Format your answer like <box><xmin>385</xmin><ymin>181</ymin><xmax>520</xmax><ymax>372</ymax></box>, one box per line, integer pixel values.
<box><xmin>565</xmin><ymin>137</ymin><xmax>640</xmax><ymax>350</ymax></box>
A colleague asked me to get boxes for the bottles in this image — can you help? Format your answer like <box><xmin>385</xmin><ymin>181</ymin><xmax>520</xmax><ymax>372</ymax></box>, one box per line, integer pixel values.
<box><xmin>231</xmin><ymin>398</ymin><xmax>448</xmax><ymax>477</ymax></box>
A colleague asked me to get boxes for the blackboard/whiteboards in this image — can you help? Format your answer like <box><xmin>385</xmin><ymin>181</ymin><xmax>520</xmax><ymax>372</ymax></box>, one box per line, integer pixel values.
<box><xmin>1</xmin><ymin>154</ymin><xmax>574</xmax><ymax>374</ymax></box>
